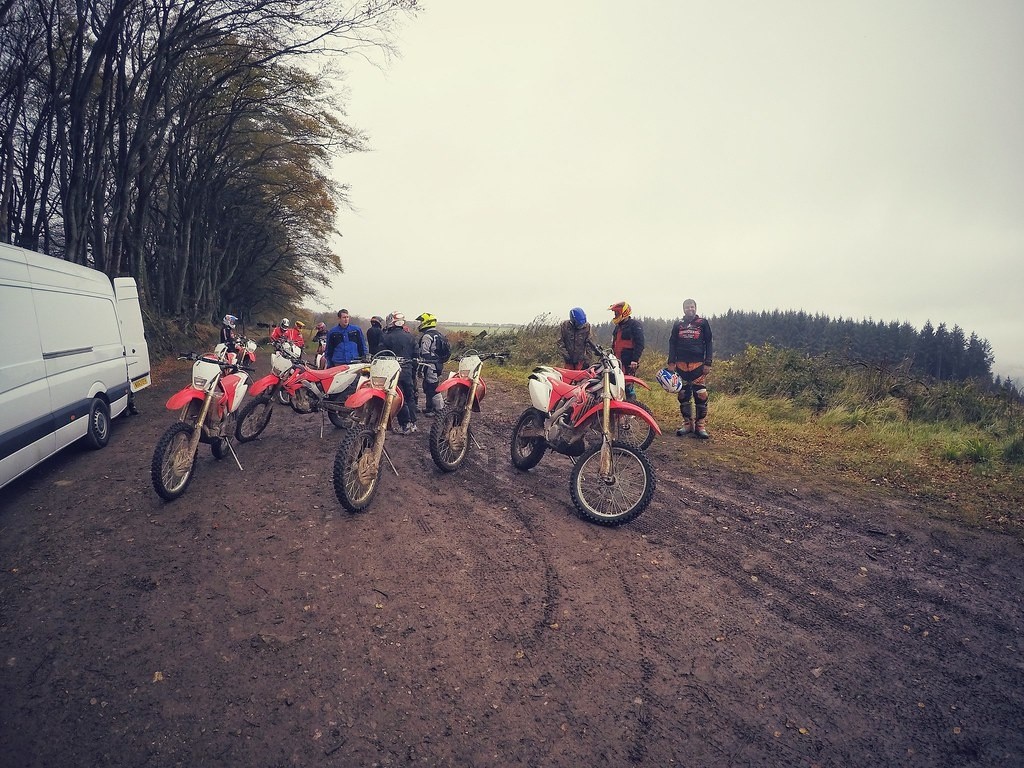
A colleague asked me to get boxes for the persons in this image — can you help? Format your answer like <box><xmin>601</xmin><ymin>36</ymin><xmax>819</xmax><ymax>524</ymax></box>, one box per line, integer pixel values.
<box><xmin>557</xmin><ymin>308</ymin><xmax>592</xmax><ymax>371</ymax></box>
<box><xmin>220</xmin><ymin>314</ymin><xmax>238</xmax><ymax>343</ymax></box>
<box><xmin>324</xmin><ymin>309</ymin><xmax>368</xmax><ymax>369</ymax></box>
<box><xmin>667</xmin><ymin>299</ymin><xmax>712</xmax><ymax>439</ymax></box>
<box><xmin>415</xmin><ymin>313</ymin><xmax>450</xmax><ymax>416</ymax></box>
<box><xmin>312</xmin><ymin>322</ymin><xmax>328</xmax><ymax>342</ymax></box>
<box><xmin>271</xmin><ymin>318</ymin><xmax>304</xmax><ymax>348</ymax></box>
<box><xmin>608</xmin><ymin>302</ymin><xmax>645</xmax><ymax>419</ymax></box>
<box><xmin>367</xmin><ymin>312</ymin><xmax>421</xmax><ymax>435</ymax></box>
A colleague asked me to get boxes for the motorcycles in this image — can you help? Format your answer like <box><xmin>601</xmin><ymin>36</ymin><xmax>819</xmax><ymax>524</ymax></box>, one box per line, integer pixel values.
<box><xmin>150</xmin><ymin>351</ymin><xmax>257</xmax><ymax>501</ymax></box>
<box><xmin>509</xmin><ymin>338</ymin><xmax>664</xmax><ymax>527</ymax></box>
<box><xmin>234</xmin><ymin>336</ymin><xmax>371</xmax><ymax>442</ymax></box>
<box><xmin>429</xmin><ymin>349</ymin><xmax>509</xmax><ymax>472</ymax></box>
<box><xmin>315</xmin><ymin>336</ymin><xmax>329</xmax><ymax>371</ymax></box>
<box><xmin>531</xmin><ymin>339</ymin><xmax>656</xmax><ymax>457</ymax></box>
<box><xmin>213</xmin><ymin>341</ymin><xmax>237</xmax><ymax>365</ymax></box>
<box><xmin>267</xmin><ymin>335</ymin><xmax>307</xmax><ymax>405</ymax></box>
<box><xmin>333</xmin><ymin>349</ymin><xmax>440</xmax><ymax>512</ymax></box>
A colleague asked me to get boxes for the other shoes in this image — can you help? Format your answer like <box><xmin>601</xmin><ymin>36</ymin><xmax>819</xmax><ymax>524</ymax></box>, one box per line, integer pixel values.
<box><xmin>403</xmin><ymin>421</ymin><xmax>417</xmax><ymax>435</ymax></box>
<box><xmin>392</xmin><ymin>427</ymin><xmax>403</xmax><ymax>434</ymax></box>
<box><xmin>422</xmin><ymin>408</ymin><xmax>438</xmax><ymax>417</ymax></box>
<box><xmin>416</xmin><ymin>407</ymin><xmax>421</xmax><ymax>413</ymax></box>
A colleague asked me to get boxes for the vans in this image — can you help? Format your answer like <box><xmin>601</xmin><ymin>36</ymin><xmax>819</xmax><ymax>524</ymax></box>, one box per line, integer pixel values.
<box><xmin>0</xmin><ymin>240</ymin><xmax>152</xmax><ymax>489</ymax></box>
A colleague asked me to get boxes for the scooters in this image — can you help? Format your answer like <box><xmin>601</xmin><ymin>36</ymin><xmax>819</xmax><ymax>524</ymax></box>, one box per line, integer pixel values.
<box><xmin>230</xmin><ymin>333</ymin><xmax>260</xmax><ymax>368</ymax></box>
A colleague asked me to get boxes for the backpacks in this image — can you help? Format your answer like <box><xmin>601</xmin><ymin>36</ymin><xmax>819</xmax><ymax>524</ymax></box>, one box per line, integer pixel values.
<box><xmin>436</xmin><ymin>333</ymin><xmax>451</xmax><ymax>364</ymax></box>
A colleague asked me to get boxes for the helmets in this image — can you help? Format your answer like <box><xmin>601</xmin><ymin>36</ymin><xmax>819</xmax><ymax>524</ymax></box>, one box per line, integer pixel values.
<box><xmin>295</xmin><ymin>321</ymin><xmax>306</xmax><ymax>333</ymax></box>
<box><xmin>281</xmin><ymin>317</ymin><xmax>290</xmax><ymax>331</ymax></box>
<box><xmin>386</xmin><ymin>311</ymin><xmax>404</xmax><ymax>329</ymax></box>
<box><xmin>371</xmin><ymin>315</ymin><xmax>384</xmax><ymax>330</ymax></box>
<box><xmin>223</xmin><ymin>315</ymin><xmax>238</xmax><ymax>329</ymax></box>
<box><xmin>317</xmin><ymin>322</ymin><xmax>326</xmax><ymax>331</ymax></box>
<box><xmin>415</xmin><ymin>313</ymin><xmax>437</xmax><ymax>332</ymax></box>
<box><xmin>569</xmin><ymin>307</ymin><xmax>587</xmax><ymax>329</ymax></box>
<box><xmin>607</xmin><ymin>302</ymin><xmax>632</xmax><ymax>325</ymax></box>
<box><xmin>655</xmin><ymin>367</ymin><xmax>683</xmax><ymax>393</ymax></box>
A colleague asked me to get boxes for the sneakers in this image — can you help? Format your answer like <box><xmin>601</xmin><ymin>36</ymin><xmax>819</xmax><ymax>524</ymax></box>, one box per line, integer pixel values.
<box><xmin>695</xmin><ymin>420</ymin><xmax>709</xmax><ymax>439</ymax></box>
<box><xmin>676</xmin><ymin>419</ymin><xmax>696</xmax><ymax>435</ymax></box>
